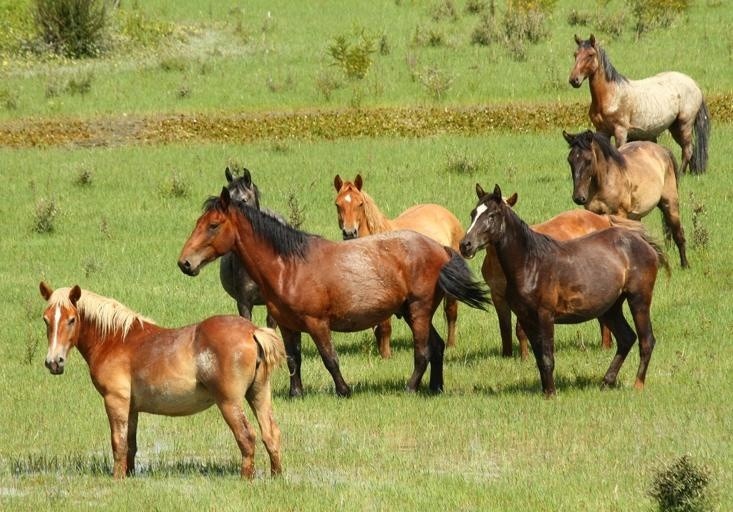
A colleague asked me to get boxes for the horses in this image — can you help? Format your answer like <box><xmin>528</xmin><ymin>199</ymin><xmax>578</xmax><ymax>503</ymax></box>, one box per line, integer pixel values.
<box><xmin>332</xmin><ymin>173</ymin><xmax>466</xmax><ymax>359</ymax></box>
<box><xmin>218</xmin><ymin>167</ymin><xmax>289</xmax><ymax>330</ymax></box>
<box><xmin>459</xmin><ymin>182</ymin><xmax>673</xmax><ymax>400</ymax></box>
<box><xmin>562</xmin><ymin>130</ymin><xmax>691</xmax><ymax>271</ymax></box>
<box><xmin>482</xmin><ymin>193</ymin><xmax>648</xmax><ymax>363</ymax></box>
<box><xmin>39</xmin><ymin>281</ymin><xmax>297</xmax><ymax>482</ymax></box>
<box><xmin>568</xmin><ymin>33</ymin><xmax>710</xmax><ymax>179</ymax></box>
<box><xmin>177</xmin><ymin>186</ymin><xmax>495</xmax><ymax>403</ymax></box>
